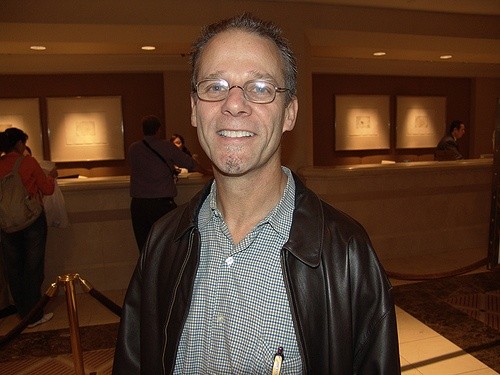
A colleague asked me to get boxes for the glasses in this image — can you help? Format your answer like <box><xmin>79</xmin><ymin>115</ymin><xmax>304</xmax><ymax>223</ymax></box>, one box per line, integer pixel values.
<box><xmin>192</xmin><ymin>77</ymin><xmax>290</xmax><ymax>104</ymax></box>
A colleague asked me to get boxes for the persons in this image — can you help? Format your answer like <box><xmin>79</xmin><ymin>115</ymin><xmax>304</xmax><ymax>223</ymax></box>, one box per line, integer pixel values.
<box><xmin>0</xmin><ymin>127</ymin><xmax>58</xmax><ymax>328</ymax></box>
<box><xmin>112</xmin><ymin>13</ymin><xmax>402</xmax><ymax>375</ymax></box>
<box><xmin>129</xmin><ymin>117</ymin><xmax>197</xmax><ymax>255</ymax></box>
<box><xmin>434</xmin><ymin>120</ymin><xmax>465</xmax><ymax>160</ymax></box>
<box><xmin>170</xmin><ymin>134</ymin><xmax>194</xmax><ymax>173</ymax></box>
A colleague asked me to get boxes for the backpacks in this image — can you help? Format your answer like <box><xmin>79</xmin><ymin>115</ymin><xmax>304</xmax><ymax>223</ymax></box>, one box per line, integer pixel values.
<box><xmin>0</xmin><ymin>155</ymin><xmax>42</xmax><ymax>232</ymax></box>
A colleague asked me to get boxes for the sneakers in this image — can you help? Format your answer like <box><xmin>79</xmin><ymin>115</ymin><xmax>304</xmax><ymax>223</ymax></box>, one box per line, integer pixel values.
<box><xmin>27</xmin><ymin>312</ymin><xmax>54</xmax><ymax>329</ymax></box>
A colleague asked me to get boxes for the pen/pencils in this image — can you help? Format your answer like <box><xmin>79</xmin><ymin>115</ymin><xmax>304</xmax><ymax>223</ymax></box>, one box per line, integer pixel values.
<box><xmin>271</xmin><ymin>347</ymin><xmax>284</xmax><ymax>375</ymax></box>
